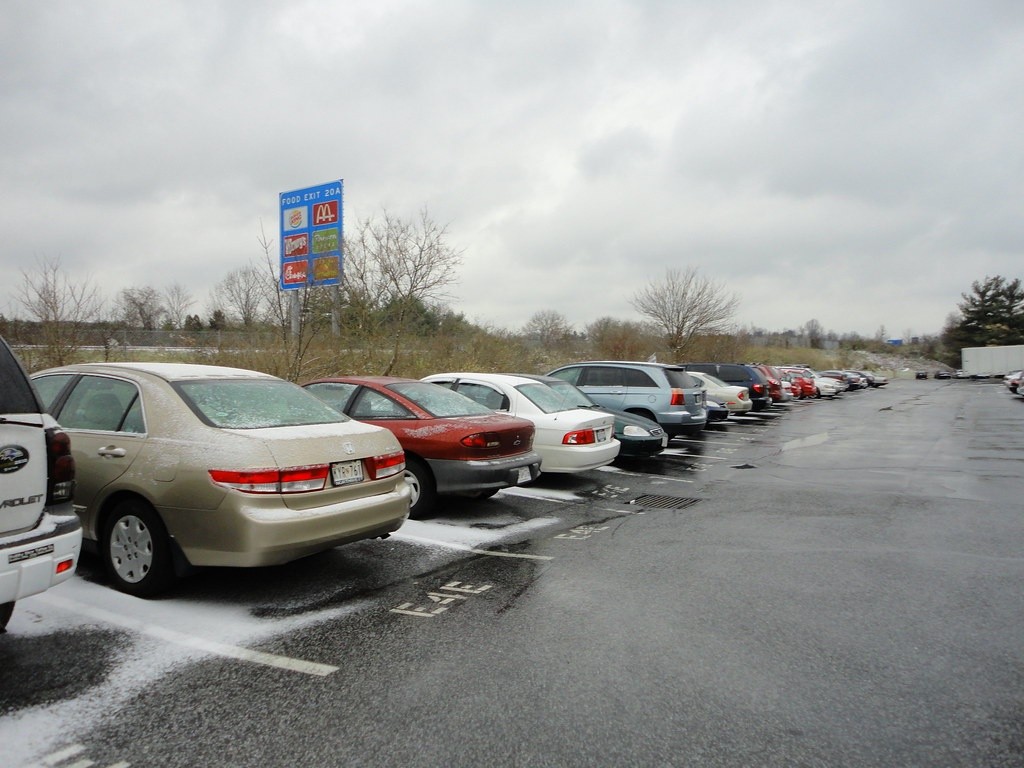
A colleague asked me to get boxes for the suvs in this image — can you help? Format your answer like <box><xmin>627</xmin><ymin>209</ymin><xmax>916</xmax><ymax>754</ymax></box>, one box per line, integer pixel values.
<box><xmin>0</xmin><ymin>333</ymin><xmax>83</xmax><ymax>636</ymax></box>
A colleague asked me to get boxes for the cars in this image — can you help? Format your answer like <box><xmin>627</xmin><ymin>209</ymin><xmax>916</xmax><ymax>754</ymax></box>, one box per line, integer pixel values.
<box><xmin>952</xmin><ymin>370</ymin><xmax>969</xmax><ymax>379</ymax></box>
<box><xmin>419</xmin><ymin>372</ymin><xmax>621</xmax><ymax>474</ymax></box>
<box><xmin>686</xmin><ymin>371</ymin><xmax>752</xmax><ymax>417</ymax></box>
<box><xmin>1002</xmin><ymin>370</ymin><xmax>1024</xmax><ymax>397</ymax></box>
<box><xmin>915</xmin><ymin>369</ymin><xmax>929</xmax><ymax>379</ymax></box>
<box><xmin>678</xmin><ymin>363</ymin><xmax>772</xmax><ymax>411</ymax></box>
<box><xmin>706</xmin><ymin>394</ymin><xmax>729</xmax><ymax>420</ymax></box>
<box><xmin>542</xmin><ymin>360</ymin><xmax>707</xmax><ymax>440</ymax></box>
<box><xmin>25</xmin><ymin>360</ymin><xmax>412</xmax><ymax>594</ymax></box>
<box><xmin>746</xmin><ymin>362</ymin><xmax>890</xmax><ymax>403</ymax></box>
<box><xmin>499</xmin><ymin>373</ymin><xmax>668</xmax><ymax>457</ymax></box>
<box><xmin>295</xmin><ymin>375</ymin><xmax>542</xmax><ymax>519</ymax></box>
<box><xmin>934</xmin><ymin>369</ymin><xmax>951</xmax><ymax>379</ymax></box>
